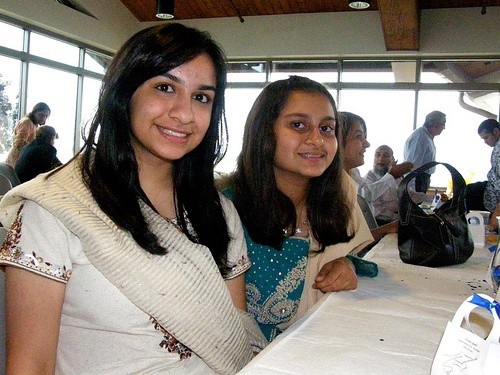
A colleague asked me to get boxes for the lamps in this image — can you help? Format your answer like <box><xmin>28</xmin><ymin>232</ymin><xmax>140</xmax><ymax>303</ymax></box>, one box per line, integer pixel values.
<box><xmin>155</xmin><ymin>0</ymin><xmax>175</xmax><ymax>20</ymax></box>
<box><xmin>348</xmin><ymin>0</ymin><xmax>371</xmax><ymax>10</ymax></box>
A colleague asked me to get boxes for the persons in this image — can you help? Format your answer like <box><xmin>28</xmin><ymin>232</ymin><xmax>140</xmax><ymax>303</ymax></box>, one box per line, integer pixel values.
<box><xmin>364</xmin><ymin>144</ymin><xmax>415</xmax><ymax>228</ymax></box>
<box><xmin>476</xmin><ymin>119</ymin><xmax>500</xmax><ymax>227</ymax></box>
<box><xmin>3</xmin><ymin>100</ymin><xmax>52</xmax><ymax>167</ymax></box>
<box><xmin>403</xmin><ymin>111</ymin><xmax>446</xmax><ymax>202</ymax></box>
<box><xmin>214</xmin><ymin>74</ymin><xmax>376</xmax><ymax>353</ymax></box>
<box><xmin>13</xmin><ymin>125</ymin><xmax>65</xmax><ymax>185</ymax></box>
<box><xmin>337</xmin><ymin>111</ymin><xmax>402</xmax><ymax>246</ymax></box>
<box><xmin>1</xmin><ymin>24</ymin><xmax>264</xmax><ymax>375</ymax></box>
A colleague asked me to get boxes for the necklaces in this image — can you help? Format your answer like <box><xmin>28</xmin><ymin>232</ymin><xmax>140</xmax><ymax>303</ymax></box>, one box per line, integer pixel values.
<box><xmin>278</xmin><ymin>199</ymin><xmax>307</xmax><ymax>235</ymax></box>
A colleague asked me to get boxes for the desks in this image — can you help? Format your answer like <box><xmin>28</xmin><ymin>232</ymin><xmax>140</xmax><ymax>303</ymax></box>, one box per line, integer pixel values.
<box><xmin>234</xmin><ymin>231</ymin><xmax>500</xmax><ymax>375</ymax></box>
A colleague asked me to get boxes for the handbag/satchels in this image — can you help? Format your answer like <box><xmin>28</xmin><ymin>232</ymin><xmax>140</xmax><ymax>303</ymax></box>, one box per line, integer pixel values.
<box><xmin>397</xmin><ymin>161</ymin><xmax>474</xmax><ymax>268</ymax></box>
